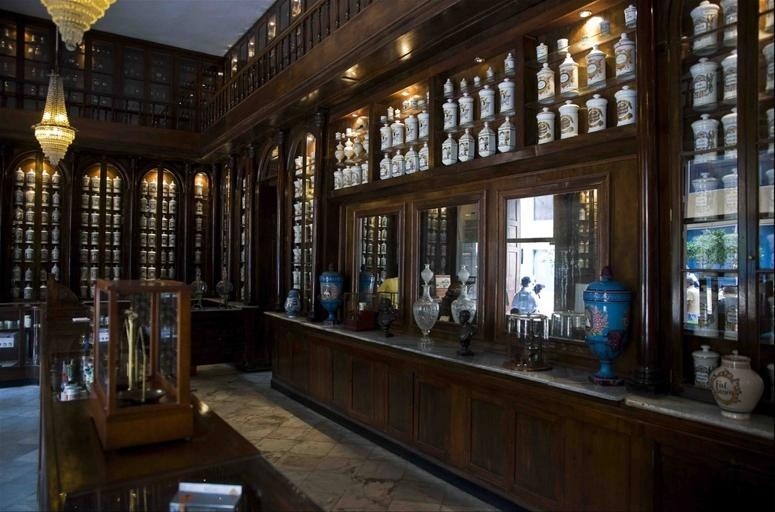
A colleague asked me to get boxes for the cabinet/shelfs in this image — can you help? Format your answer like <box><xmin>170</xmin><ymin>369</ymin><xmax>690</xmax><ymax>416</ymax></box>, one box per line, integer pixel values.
<box><xmin>262</xmin><ymin>310</ymin><xmax>475</xmax><ymax>483</ymax></box>
<box><xmin>0</xmin><ymin>1</ymin><xmax>464</xmax><ymax>377</ymax></box>
<box><xmin>463</xmin><ymin>0</ymin><xmax>775</xmax><ymax>511</ymax></box>
<box><xmin>37</xmin><ymin>375</ymin><xmax>330</xmax><ymax>510</ymax></box>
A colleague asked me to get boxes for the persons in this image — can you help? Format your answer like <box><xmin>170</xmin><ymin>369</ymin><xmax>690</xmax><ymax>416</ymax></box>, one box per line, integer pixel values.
<box><xmin>687</xmin><ymin>276</ymin><xmax>737</xmax><ymax>332</ymax></box>
<box><xmin>511</xmin><ymin>276</ymin><xmax>537</xmax><ymax>312</ymax></box>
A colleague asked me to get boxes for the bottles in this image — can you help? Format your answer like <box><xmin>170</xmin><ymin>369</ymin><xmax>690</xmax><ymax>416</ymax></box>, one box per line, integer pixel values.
<box><xmin>677</xmin><ymin>1</ymin><xmax>775</xmax><ymax>353</ymax></box>
<box><xmin>582</xmin><ymin>266</ymin><xmax>633</xmax><ymax>388</ymax></box>
<box><xmin>357</xmin><ymin>263</ymin><xmax>374</xmax><ymax>303</ymax></box>
<box><xmin>0</xmin><ymin>314</ymin><xmax>32</xmax><ymax>330</ymax></box>
<box><xmin>422</xmin><ymin>207</ymin><xmax>450</xmax><ymax>279</ymax></box>
<box><xmin>318</xmin><ymin>262</ymin><xmax>343</xmax><ymax>326</ymax></box>
<box><xmin>284</xmin><ymin>289</ymin><xmax>300</xmax><ymax>319</ymax></box>
<box><xmin>412</xmin><ymin>263</ymin><xmax>439</xmax><ymax>344</ymax></box>
<box><xmin>692</xmin><ymin>344</ymin><xmax>719</xmax><ymax>389</ymax></box>
<box><xmin>79</xmin><ymin>173</ymin><xmax>125</xmax><ymax>301</ymax></box>
<box><xmin>99</xmin><ymin>315</ymin><xmax>110</xmax><ymax>343</ymax></box>
<box><xmin>195</xmin><ymin>179</ymin><xmax>203</xmax><ymax>280</ymax></box>
<box><xmin>241</xmin><ymin>179</ymin><xmax>246</xmax><ymax>301</ymax></box>
<box><xmin>708</xmin><ymin>350</ymin><xmax>767</xmax><ymax>421</ymax></box>
<box><xmin>357</xmin><ymin>215</ymin><xmax>389</xmax><ymax>292</ymax></box>
<box><xmin>140</xmin><ymin>177</ymin><xmax>179</xmax><ymax>286</ymax></box>
<box><xmin>291</xmin><ymin>149</ymin><xmax>315</xmax><ymax>293</ymax></box>
<box><xmin>333</xmin><ymin>0</ymin><xmax>639</xmax><ymax>189</ymax></box>
<box><xmin>449</xmin><ymin>263</ymin><xmax>478</xmax><ymax>326</ymax></box>
<box><xmin>12</xmin><ymin>165</ymin><xmax>61</xmax><ymax>301</ymax></box>
<box><xmin>59</xmin><ymin>357</ymin><xmax>96</xmax><ymax>402</ymax></box>
<box><xmin>577</xmin><ymin>188</ymin><xmax>591</xmax><ymax>269</ymax></box>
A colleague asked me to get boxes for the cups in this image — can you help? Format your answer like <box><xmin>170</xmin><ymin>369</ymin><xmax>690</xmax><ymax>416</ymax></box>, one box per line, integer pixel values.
<box><xmin>552</xmin><ymin>311</ymin><xmax>584</xmax><ymax>338</ymax></box>
<box><xmin>505</xmin><ymin>313</ymin><xmax>550</xmax><ymax>342</ymax></box>
